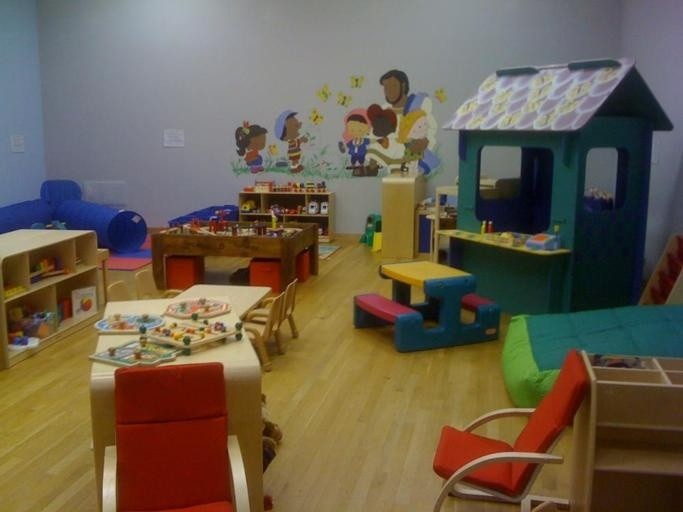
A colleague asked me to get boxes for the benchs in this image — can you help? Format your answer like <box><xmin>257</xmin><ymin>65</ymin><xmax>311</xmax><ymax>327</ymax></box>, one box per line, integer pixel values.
<box><xmin>354</xmin><ymin>293</ymin><xmax>444</xmax><ymax>352</ymax></box>
<box><xmin>460</xmin><ymin>294</ymin><xmax>502</xmax><ymax>343</ymax></box>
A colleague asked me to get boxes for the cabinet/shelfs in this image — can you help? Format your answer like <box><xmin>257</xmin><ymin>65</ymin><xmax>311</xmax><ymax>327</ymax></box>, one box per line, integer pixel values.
<box><xmin>90</xmin><ymin>301</ymin><xmax>266</xmax><ymax>511</ymax></box>
<box><xmin>572</xmin><ymin>349</ymin><xmax>683</xmax><ymax>512</ymax></box>
<box><xmin>236</xmin><ymin>191</ymin><xmax>335</xmax><ymax>243</ymax></box>
<box><xmin>636</xmin><ymin>233</ymin><xmax>682</xmax><ymax>307</ymax></box>
<box><xmin>0</xmin><ymin>228</ymin><xmax>104</xmax><ymax>368</ymax></box>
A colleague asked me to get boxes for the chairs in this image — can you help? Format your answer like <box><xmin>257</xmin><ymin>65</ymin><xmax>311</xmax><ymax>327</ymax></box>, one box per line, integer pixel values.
<box><xmin>102</xmin><ymin>362</ymin><xmax>249</xmax><ymax>512</ymax></box>
<box><xmin>136</xmin><ymin>271</ymin><xmax>180</xmax><ymax>297</ymax></box>
<box><xmin>106</xmin><ymin>280</ymin><xmax>130</xmax><ymax>301</ymax></box>
<box><xmin>243</xmin><ymin>293</ymin><xmax>284</xmax><ymax>373</ymax></box>
<box><xmin>251</xmin><ymin>279</ymin><xmax>298</xmax><ymax>339</ymax></box>
<box><xmin>432</xmin><ymin>348</ymin><xmax>592</xmax><ymax>511</ymax></box>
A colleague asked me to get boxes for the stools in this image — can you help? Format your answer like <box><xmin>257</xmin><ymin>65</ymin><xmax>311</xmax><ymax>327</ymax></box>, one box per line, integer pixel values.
<box><xmin>166</xmin><ymin>251</ymin><xmax>311</xmax><ymax>294</ymax></box>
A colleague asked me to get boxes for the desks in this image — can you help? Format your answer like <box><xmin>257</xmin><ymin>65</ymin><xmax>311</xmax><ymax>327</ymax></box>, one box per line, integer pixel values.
<box><xmin>433</xmin><ymin>179</ymin><xmax>518</xmax><ymax>260</ymax></box>
<box><xmin>150</xmin><ymin>220</ymin><xmax>319</xmax><ymax>292</ymax></box>
<box><xmin>381</xmin><ymin>172</ymin><xmax>424</xmax><ymax>262</ymax></box>
<box><xmin>377</xmin><ymin>261</ymin><xmax>476</xmax><ymax>348</ymax></box>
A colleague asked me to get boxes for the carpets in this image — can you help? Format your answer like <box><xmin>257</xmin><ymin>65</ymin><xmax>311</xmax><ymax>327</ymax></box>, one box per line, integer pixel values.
<box><xmin>318</xmin><ymin>243</ymin><xmax>341</xmax><ymax>259</ymax></box>
